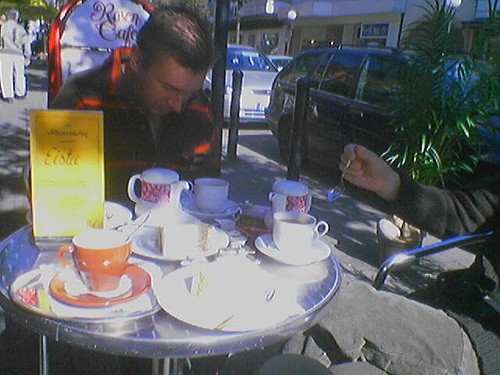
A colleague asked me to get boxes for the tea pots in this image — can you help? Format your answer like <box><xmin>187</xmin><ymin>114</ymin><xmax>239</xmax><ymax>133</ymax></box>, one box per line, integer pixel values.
<box><xmin>127</xmin><ymin>168</ymin><xmax>190</xmax><ymax>217</ymax></box>
<box><xmin>264</xmin><ymin>180</ymin><xmax>313</xmax><ymax>230</ymax></box>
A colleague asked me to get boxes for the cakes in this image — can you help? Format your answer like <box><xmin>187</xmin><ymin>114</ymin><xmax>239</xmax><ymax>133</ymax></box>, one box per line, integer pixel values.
<box><xmin>155</xmin><ymin>223</ymin><xmax>209</xmax><ymax>255</ymax></box>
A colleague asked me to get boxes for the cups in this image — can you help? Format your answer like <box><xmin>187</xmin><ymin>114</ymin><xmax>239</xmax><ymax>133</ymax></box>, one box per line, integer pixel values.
<box><xmin>185</xmin><ymin>177</ymin><xmax>230</xmax><ymax>213</ymax></box>
<box><xmin>271</xmin><ymin>210</ymin><xmax>329</xmax><ymax>255</ymax></box>
<box><xmin>57</xmin><ymin>229</ymin><xmax>133</xmax><ymax>293</ymax></box>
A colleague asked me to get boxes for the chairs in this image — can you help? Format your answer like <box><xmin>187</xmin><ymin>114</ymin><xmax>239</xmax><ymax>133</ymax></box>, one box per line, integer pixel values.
<box><xmin>372</xmin><ymin>229</ymin><xmax>497</xmax><ymax>294</ymax></box>
<box><xmin>22</xmin><ymin>160</ymin><xmax>37</xmax><ymax>223</ymax></box>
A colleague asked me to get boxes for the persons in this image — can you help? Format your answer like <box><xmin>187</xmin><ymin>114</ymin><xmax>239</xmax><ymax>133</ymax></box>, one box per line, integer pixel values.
<box><xmin>0</xmin><ymin>7</ymin><xmax>32</xmax><ymax>102</ymax></box>
<box><xmin>340</xmin><ymin>144</ymin><xmax>500</xmax><ymax>283</ymax></box>
<box><xmin>49</xmin><ymin>4</ymin><xmax>222</xmax><ymax>200</ymax></box>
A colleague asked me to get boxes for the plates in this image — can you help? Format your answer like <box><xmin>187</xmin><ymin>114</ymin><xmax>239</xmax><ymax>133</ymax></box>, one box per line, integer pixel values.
<box><xmin>181</xmin><ymin>199</ymin><xmax>239</xmax><ymax>218</ymax></box>
<box><xmin>48</xmin><ymin>261</ymin><xmax>152</xmax><ymax>307</ymax></box>
<box><xmin>254</xmin><ymin>232</ymin><xmax>330</xmax><ymax>266</ymax></box>
<box><xmin>104</xmin><ymin>200</ymin><xmax>133</xmax><ymax>229</ymax></box>
<box><xmin>154</xmin><ymin>261</ymin><xmax>298</xmax><ymax>331</ymax></box>
<box><xmin>131</xmin><ymin>220</ymin><xmax>229</xmax><ymax>261</ymax></box>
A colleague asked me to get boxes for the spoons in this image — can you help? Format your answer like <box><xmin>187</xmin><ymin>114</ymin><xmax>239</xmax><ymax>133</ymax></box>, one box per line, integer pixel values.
<box><xmin>327</xmin><ymin>159</ymin><xmax>352</xmax><ymax>202</ymax></box>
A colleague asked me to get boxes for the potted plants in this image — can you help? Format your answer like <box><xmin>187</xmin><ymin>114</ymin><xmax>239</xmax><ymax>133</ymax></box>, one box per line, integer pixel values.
<box><xmin>372</xmin><ymin>2</ymin><xmax>499</xmax><ymax>272</ymax></box>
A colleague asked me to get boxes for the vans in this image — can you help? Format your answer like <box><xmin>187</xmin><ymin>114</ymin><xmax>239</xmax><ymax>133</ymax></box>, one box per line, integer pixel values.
<box><xmin>265</xmin><ymin>44</ymin><xmax>500</xmax><ymax>193</ymax></box>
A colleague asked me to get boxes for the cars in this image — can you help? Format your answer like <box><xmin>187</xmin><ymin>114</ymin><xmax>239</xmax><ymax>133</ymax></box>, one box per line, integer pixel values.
<box><xmin>206</xmin><ymin>45</ymin><xmax>279</xmax><ymax>125</ymax></box>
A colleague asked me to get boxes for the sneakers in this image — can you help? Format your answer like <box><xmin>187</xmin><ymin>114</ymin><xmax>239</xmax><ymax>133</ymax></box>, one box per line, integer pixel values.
<box><xmin>16</xmin><ymin>94</ymin><xmax>26</xmax><ymax>99</ymax></box>
<box><xmin>3</xmin><ymin>98</ymin><xmax>13</xmax><ymax>103</ymax></box>
